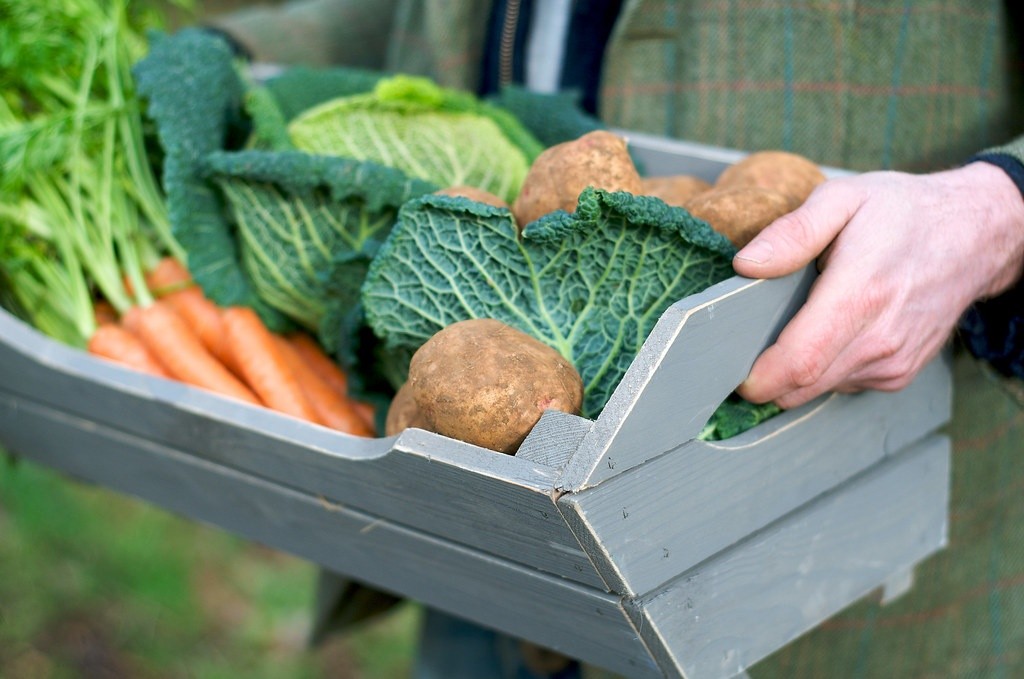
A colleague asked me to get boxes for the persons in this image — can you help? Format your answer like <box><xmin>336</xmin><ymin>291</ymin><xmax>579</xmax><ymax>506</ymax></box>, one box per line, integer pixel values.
<box><xmin>157</xmin><ymin>1</ymin><xmax>1024</xmax><ymax>679</ymax></box>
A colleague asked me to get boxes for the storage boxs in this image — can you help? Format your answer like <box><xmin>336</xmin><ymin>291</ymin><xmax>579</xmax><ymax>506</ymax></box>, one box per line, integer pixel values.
<box><xmin>1</xmin><ymin>60</ymin><xmax>955</xmax><ymax>678</ymax></box>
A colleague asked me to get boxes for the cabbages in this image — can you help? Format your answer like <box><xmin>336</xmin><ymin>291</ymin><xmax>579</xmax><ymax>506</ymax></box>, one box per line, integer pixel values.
<box><xmin>138</xmin><ymin>27</ymin><xmax>799</xmax><ymax>446</ymax></box>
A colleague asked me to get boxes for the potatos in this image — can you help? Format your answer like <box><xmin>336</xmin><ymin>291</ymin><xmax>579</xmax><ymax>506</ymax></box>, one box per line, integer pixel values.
<box><xmin>383</xmin><ymin>311</ymin><xmax>582</xmax><ymax>460</ymax></box>
<box><xmin>434</xmin><ymin>123</ymin><xmax>829</xmax><ymax>246</ymax></box>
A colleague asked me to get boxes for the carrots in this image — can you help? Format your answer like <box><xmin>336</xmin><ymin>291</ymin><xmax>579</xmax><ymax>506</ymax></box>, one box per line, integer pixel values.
<box><xmin>1</xmin><ymin>0</ymin><xmax>378</xmax><ymax>437</ymax></box>
